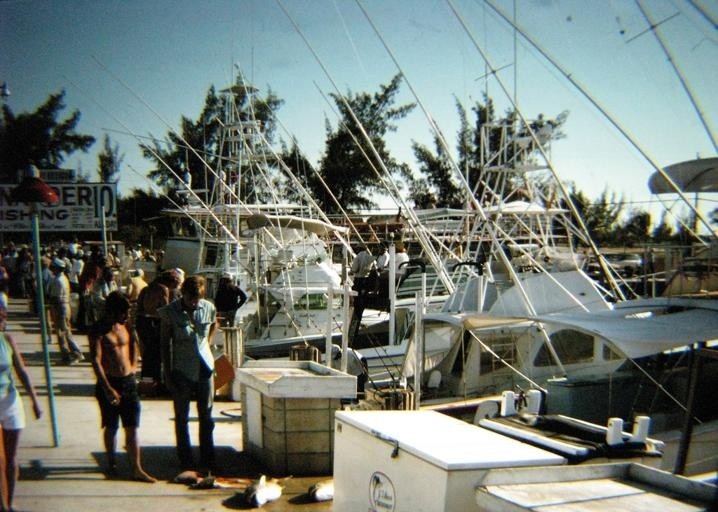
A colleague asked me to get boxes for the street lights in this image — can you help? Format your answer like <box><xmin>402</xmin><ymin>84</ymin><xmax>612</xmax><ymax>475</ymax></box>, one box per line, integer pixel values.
<box><xmin>7</xmin><ymin>165</ymin><xmax>64</xmax><ymax>448</ymax></box>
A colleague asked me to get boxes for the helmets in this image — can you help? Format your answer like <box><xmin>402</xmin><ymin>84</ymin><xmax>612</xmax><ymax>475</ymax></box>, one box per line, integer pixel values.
<box><xmin>54</xmin><ymin>258</ymin><xmax>65</xmax><ymax>269</ymax></box>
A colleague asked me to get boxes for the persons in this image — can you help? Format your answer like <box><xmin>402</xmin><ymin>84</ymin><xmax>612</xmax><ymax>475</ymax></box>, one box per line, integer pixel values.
<box><xmin>151</xmin><ymin>275</ymin><xmax>218</xmax><ymax>481</ymax></box>
<box><xmin>395</xmin><ymin>240</ymin><xmax>410</xmax><ymax>290</ymax></box>
<box><xmin>0</xmin><ymin>305</ymin><xmax>43</xmax><ymax>512</ymax></box>
<box><xmin>647</xmin><ymin>248</ymin><xmax>655</xmax><ymax>270</ymax></box>
<box><xmin>0</xmin><ymin>236</ymin><xmax>246</xmax><ymax>392</ymax></box>
<box><xmin>349</xmin><ymin>243</ymin><xmax>374</xmax><ymax>308</ymax></box>
<box><xmin>581</xmin><ymin>250</ymin><xmax>590</xmax><ymax>273</ymax></box>
<box><xmin>91</xmin><ymin>291</ymin><xmax>158</xmax><ymax>483</ymax></box>
<box><xmin>373</xmin><ymin>240</ymin><xmax>390</xmax><ymax>274</ymax></box>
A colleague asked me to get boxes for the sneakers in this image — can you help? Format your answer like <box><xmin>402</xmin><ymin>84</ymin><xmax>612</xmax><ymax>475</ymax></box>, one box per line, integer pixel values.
<box><xmin>56</xmin><ymin>356</ymin><xmax>72</xmax><ymax>365</ymax></box>
<box><xmin>68</xmin><ymin>353</ymin><xmax>86</xmax><ymax>366</ymax></box>
